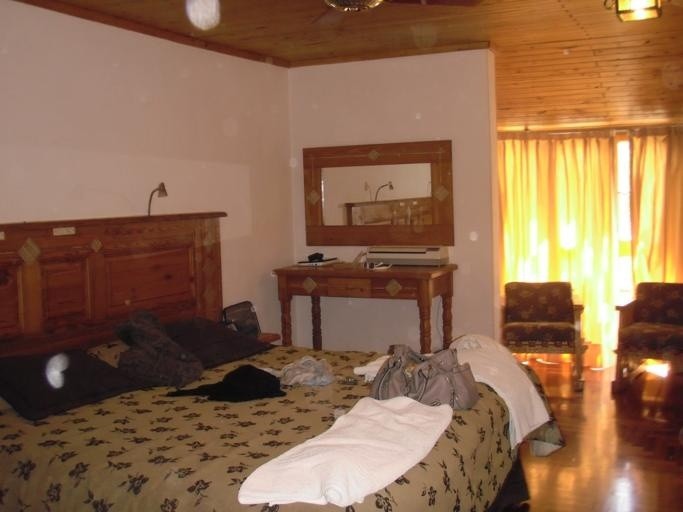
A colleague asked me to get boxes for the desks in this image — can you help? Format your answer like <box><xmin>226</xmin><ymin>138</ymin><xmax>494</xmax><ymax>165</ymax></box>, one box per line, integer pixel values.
<box><xmin>270</xmin><ymin>260</ymin><xmax>458</xmax><ymax>358</ymax></box>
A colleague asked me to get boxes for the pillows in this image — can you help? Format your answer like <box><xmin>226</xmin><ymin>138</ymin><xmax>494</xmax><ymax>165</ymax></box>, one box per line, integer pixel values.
<box><xmin>84</xmin><ymin>338</ymin><xmax>128</xmax><ymax>367</ymax></box>
<box><xmin>116</xmin><ymin>310</ymin><xmax>203</xmax><ymax>390</ymax></box>
<box><xmin>119</xmin><ymin>353</ymin><xmax>177</xmax><ymax>392</ymax></box>
<box><xmin>157</xmin><ymin>315</ymin><xmax>271</xmax><ymax>372</ymax></box>
<box><xmin>0</xmin><ymin>348</ymin><xmax>141</xmax><ymax>420</ymax></box>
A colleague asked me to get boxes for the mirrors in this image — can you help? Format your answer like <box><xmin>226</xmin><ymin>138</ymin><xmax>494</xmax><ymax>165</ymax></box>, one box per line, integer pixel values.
<box><xmin>301</xmin><ymin>139</ymin><xmax>454</xmax><ymax>247</ymax></box>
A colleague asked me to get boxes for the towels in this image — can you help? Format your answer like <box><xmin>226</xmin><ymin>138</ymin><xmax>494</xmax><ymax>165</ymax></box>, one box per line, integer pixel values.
<box><xmin>353</xmin><ymin>333</ymin><xmax>552</xmax><ymax>450</ymax></box>
<box><xmin>238</xmin><ymin>394</ymin><xmax>455</xmax><ymax>506</ymax></box>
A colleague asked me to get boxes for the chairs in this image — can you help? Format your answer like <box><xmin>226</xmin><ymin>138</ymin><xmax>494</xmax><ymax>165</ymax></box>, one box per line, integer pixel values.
<box><xmin>611</xmin><ymin>281</ymin><xmax>683</xmax><ymax>394</ymax></box>
<box><xmin>502</xmin><ymin>280</ymin><xmax>588</xmax><ymax>392</ymax></box>
<box><xmin>223</xmin><ymin>300</ymin><xmax>280</xmax><ymax>346</ymax></box>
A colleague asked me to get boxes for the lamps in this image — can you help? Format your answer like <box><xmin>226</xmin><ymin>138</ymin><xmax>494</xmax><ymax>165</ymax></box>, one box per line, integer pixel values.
<box><xmin>604</xmin><ymin>0</ymin><xmax>662</xmax><ymax>25</ymax></box>
<box><xmin>323</xmin><ymin>0</ymin><xmax>383</xmax><ymax>17</ymax></box>
<box><xmin>146</xmin><ymin>183</ymin><xmax>167</xmax><ymax>214</ymax></box>
<box><xmin>374</xmin><ymin>180</ymin><xmax>394</xmax><ymax>200</ymax></box>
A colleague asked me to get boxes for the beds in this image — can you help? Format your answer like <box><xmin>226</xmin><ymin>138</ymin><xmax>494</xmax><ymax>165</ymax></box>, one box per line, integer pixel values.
<box><xmin>0</xmin><ymin>211</ymin><xmax>564</xmax><ymax>511</ymax></box>
<box><xmin>344</xmin><ymin>197</ymin><xmax>433</xmax><ymax>226</ymax></box>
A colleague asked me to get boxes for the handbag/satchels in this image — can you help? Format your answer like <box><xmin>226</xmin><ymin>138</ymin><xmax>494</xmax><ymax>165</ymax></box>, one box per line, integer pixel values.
<box><xmin>370</xmin><ymin>348</ymin><xmax>479</xmax><ymax>410</ymax></box>
